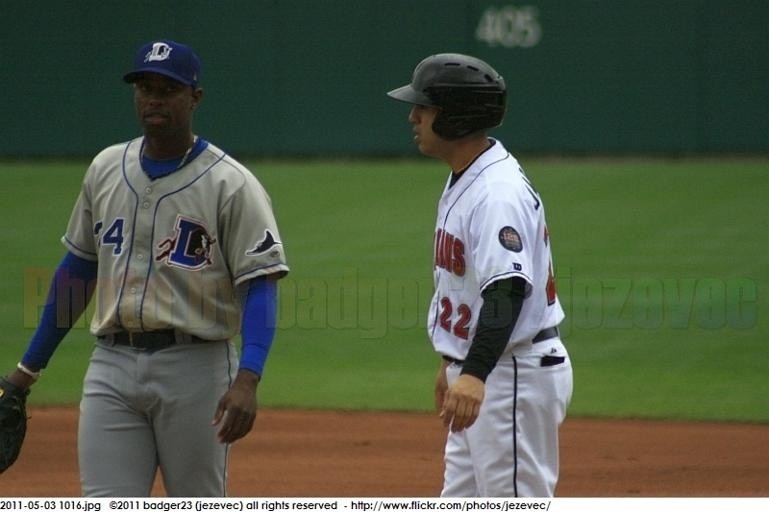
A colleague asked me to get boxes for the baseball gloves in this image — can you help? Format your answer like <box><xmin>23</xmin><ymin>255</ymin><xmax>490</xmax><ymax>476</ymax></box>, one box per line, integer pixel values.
<box><xmin>0</xmin><ymin>373</ymin><xmax>30</xmax><ymax>473</ymax></box>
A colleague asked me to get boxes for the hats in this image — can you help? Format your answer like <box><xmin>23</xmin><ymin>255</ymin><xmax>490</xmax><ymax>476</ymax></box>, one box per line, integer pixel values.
<box><xmin>122</xmin><ymin>41</ymin><xmax>202</xmax><ymax>90</ymax></box>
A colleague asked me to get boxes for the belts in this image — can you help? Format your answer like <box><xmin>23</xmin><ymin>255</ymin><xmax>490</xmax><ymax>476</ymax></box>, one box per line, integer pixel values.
<box><xmin>96</xmin><ymin>331</ymin><xmax>204</xmax><ymax>351</ymax></box>
<box><xmin>442</xmin><ymin>325</ymin><xmax>559</xmax><ymax>365</ymax></box>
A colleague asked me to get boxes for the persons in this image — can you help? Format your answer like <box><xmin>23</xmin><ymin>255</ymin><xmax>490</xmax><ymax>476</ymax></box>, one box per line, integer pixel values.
<box><xmin>0</xmin><ymin>39</ymin><xmax>289</xmax><ymax>498</ymax></box>
<box><xmin>386</xmin><ymin>54</ymin><xmax>573</xmax><ymax>496</ymax></box>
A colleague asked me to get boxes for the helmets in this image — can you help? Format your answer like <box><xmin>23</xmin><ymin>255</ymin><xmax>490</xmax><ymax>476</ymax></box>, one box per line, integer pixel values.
<box><xmin>385</xmin><ymin>52</ymin><xmax>508</xmax><ymax>143</ymax></box>
